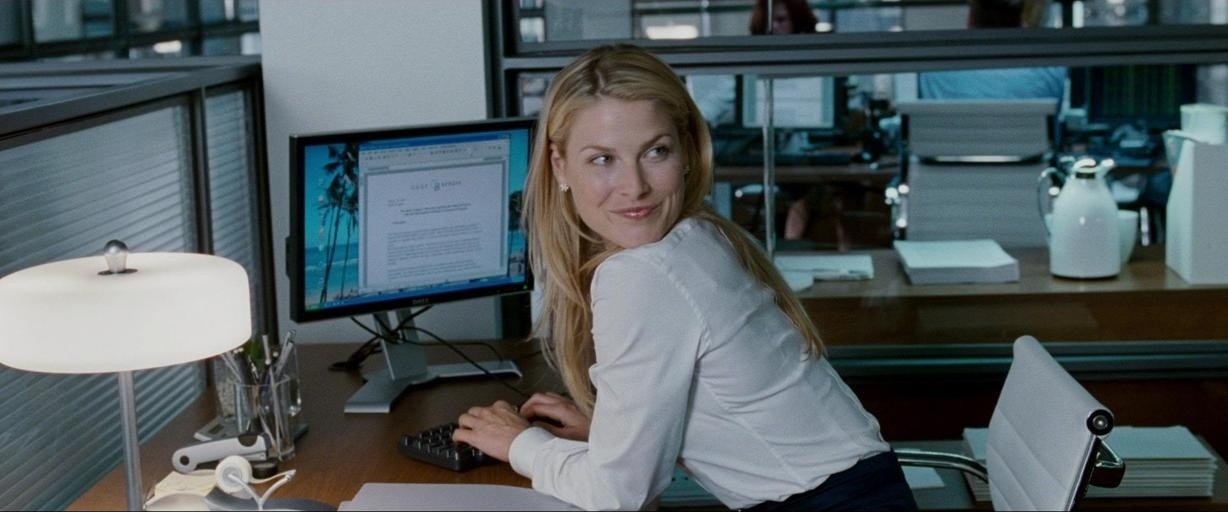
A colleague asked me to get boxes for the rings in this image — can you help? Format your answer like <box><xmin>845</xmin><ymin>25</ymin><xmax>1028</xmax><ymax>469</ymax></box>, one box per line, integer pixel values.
<box><xmin>222</xmin><ymin>330</ymin><xmax>294</xmax><ymax>454</ymax></box>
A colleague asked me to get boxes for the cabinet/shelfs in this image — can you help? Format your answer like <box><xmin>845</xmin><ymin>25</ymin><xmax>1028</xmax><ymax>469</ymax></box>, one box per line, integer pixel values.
<box><xmin>235</xmin><ymin>379</ymin><xmax>298</xmax><ymax>467</ymax></box>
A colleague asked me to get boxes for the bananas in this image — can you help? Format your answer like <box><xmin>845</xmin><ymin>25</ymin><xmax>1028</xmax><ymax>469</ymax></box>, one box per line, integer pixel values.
<box><xmin>715</xmin><ymin>151</ymin><xmax>852</xmax><ymax>168</ymax></box>
<box><xmin>397</xmin><ymin>391</ymin><xmax>573</xmax><ymax>471</ymax></box>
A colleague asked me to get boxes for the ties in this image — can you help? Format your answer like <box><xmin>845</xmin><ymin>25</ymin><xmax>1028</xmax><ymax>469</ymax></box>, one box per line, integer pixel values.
<box><xmin>284</xmin><ymin>116</ymin><xmax>541</xmax><ymax>415</ymax></box>
<box><xmin>734</xmin><ymin>75</ymin><xmax>842</xmax><ymax>151</ymax></box>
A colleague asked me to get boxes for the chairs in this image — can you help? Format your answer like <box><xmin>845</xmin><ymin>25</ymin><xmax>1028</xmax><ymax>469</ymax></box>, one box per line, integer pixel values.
<box><xmin>896</xmin><ymin>335</ymin><xmax>1128</xmax><ymax>512</ymax></box>
<box><xmin>880</xmin><ymin>97</ymin><xmax>1062</xmax><ymax>248</ymax></box>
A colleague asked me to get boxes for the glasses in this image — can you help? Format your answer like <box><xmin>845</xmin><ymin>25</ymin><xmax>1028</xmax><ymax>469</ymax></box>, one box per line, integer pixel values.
<box><xmin>3</xmin><ymin>238</ymin><xmax>254</xmax><ymax>512</ymax></box>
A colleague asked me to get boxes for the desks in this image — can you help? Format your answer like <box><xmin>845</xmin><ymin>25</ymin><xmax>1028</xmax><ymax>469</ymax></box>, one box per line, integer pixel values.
<box><xmin>713</xmin><ymin>247</ymin><xmax>1227</xmax><ymax>457</ymax></box>
<box><xmin>67</xmin><ymin>335</ymin><xmax>599</xmax><ymax>512</ymax></box>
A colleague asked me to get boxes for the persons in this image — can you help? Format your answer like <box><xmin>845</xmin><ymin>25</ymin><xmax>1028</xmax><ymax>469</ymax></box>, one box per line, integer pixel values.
<box><xmin>919</xmin><ymin>0</ymin><xmax>1064</xmax><ymax>116</ymax></box>
<box><xmin>450</xmin><ymin>42</ymin><xmax>918</xmax><ymax>512</ymax></box>
<box><xmin>696</xmin><ymin>0</ymin><xmax>819</xmax><ymax>128</ymax></box>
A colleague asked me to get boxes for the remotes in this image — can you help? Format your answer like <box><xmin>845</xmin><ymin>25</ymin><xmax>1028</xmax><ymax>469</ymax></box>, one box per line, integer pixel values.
<box><xmin>854</xmin><ymin>150</ymin><xmax>873</xmax><ymax>164</ymax></box>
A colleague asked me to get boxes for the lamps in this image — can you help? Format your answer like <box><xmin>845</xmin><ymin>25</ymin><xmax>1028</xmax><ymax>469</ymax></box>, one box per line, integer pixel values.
<box><xmin>3</xmin><ymin>238</ymin><xmax>254</xmax><ymax>512</ymax></box>
<box><xmin>896</xmin><ymin>335</ymin><xmax>1128</xmax><ymax>512</ymax></box>
<box><xmin>880</xmin><ymin>97</ymin><xmax>1062</xmax><ymax>248</ymax></box>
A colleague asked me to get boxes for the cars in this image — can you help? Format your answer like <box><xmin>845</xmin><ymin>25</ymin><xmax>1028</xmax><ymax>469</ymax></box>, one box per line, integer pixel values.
<box><xmin>1120</xmin><ymin>206</ymin><xmax>1141</xmax><ymax>265</ymax></box>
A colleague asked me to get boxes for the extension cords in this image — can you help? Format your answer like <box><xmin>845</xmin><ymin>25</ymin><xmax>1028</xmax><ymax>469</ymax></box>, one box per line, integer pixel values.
<box><xmin>1035</xmin><ymin>159</ymin><xmax>1124</xmax><ymax>279</ymax></box>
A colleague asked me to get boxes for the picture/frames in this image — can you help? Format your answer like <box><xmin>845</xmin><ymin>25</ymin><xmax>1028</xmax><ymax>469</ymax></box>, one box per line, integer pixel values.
<box><xmin>67</xmin><ymin>335</ymin><xmax>599</xmax><ymax>512</ymax></box>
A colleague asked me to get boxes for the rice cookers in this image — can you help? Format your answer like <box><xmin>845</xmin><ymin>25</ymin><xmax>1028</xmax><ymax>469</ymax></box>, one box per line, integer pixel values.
<box><xmin>171</xmin><ymin>431</ymin><xmax>280</xmax><ymax>481</ymax></box>
